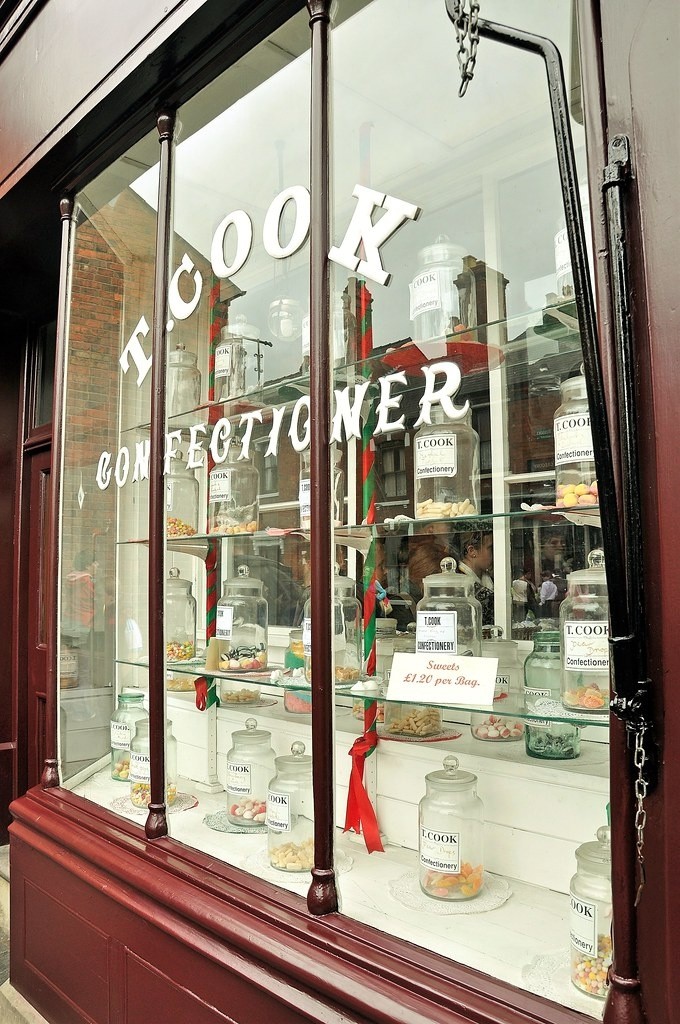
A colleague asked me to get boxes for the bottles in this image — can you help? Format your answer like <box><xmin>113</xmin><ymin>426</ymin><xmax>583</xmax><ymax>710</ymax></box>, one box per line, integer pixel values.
<box><xmin>164</xmin><ymin>568</ymin><xmax>197</xmax><ymax>663</ymax></box>
<box><xmin>61</xmin><ymin>623</ymin><xmax>80</xmax><ymax>691</ymax></box>
<box><xmin>571</xmin><ymin>827</ymin><xmax>614</xmax><ymax>999</ymax></box>
<box><xmin>190</xmin><ymin>180</ymin><xmax>609</xmax><ymax>760</ymax></box>
<box><xmin>226</xmin><ymin>718</ymin><xmax>275</xmax><ymax>828</ymax></box>
<box><xmin>110</xmin><ymin>691</ymin><xmax>149</xmax><ymax>782</ymax></box>
<box><xmin>129</xmin><ymin>719</ymin><xmax>176</xmax><ymax>808</ymax></box>
<box><xmin>167</xmin><ymin>450</ymin><xmax>199</xmax><ymax>539</ymax></box>
<box><xmin>167</xmin><ymin>343</ymin><xmax>201</xmax><ymax>427</ymax></box>
<box><xmin>416</xmin><ymin>757</ymin><xmax>484</xmax><ymax>900</ymax></box>
<box><xmin>268</xmin><ymin>742</ymin><xmax>315</xmax><ymax>874</ymax></box>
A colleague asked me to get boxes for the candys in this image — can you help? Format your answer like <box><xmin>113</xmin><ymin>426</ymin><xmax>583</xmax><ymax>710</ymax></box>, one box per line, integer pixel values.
<box><xmin>112</xmin><ymin>482</ymin><xmax>616</xmax><ymax>1001</ymax></box>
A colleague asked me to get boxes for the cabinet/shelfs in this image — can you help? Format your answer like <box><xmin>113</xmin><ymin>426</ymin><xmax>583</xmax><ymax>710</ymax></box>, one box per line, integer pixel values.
<box><xmin>72</xmin><ymin>621</ymin><xmax>614</xmax><ymax>1022</ymax></box>
<box><xmin>112</xmin><ymin>290</ymin><xmax>611</xmax><ymax>727</ymax></box>
